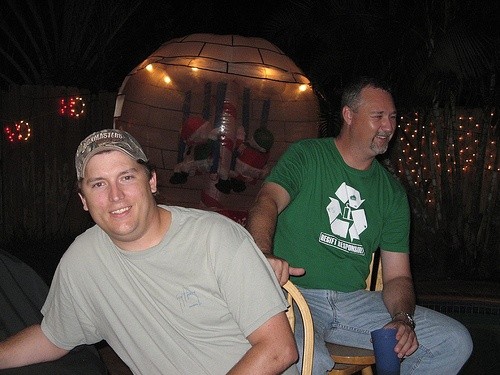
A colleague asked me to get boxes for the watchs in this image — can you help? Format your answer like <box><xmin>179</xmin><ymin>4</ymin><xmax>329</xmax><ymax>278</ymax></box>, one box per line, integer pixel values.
<box><xmin>392</xmin><ymin>311</ymin><xmax>414</xmax><ymax>327</ymax></box>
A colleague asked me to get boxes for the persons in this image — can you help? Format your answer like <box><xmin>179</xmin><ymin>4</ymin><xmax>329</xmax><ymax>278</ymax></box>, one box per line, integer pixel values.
<box><xmin>1</xmin><ymin>130</ymin><xmax>301</xmax><ymax>375</ymax></box>
<box><xmin>247</xmin><ymin>79</ymin><xmax>473</xmax><ymax>375</ymax></box>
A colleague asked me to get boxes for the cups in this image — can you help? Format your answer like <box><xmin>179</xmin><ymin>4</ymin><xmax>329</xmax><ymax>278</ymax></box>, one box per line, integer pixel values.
<box><xmin>371</xmin><ymin>327</ymin><xmax>401</xmax><ymax>375</ymax></box>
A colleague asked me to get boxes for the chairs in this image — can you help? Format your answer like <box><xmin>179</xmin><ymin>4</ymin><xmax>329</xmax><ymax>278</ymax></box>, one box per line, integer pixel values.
<box><xmin>279</xmin><ymin>279</ymin><xmax>314</xmax><ymax>375</ymax></box>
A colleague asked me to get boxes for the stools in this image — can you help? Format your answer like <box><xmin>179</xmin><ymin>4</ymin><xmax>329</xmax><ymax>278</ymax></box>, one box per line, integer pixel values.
<box><xmin>323</xmin><ymin>342</ymin><xmax>376</xmax><ymax>375</ymax></box>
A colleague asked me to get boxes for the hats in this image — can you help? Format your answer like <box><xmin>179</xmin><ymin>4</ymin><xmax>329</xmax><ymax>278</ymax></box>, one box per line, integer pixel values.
<box><xmin>75</xmin><ymin>129</ymin><xmax>148</xmax><ymax>181</ymax></box>
